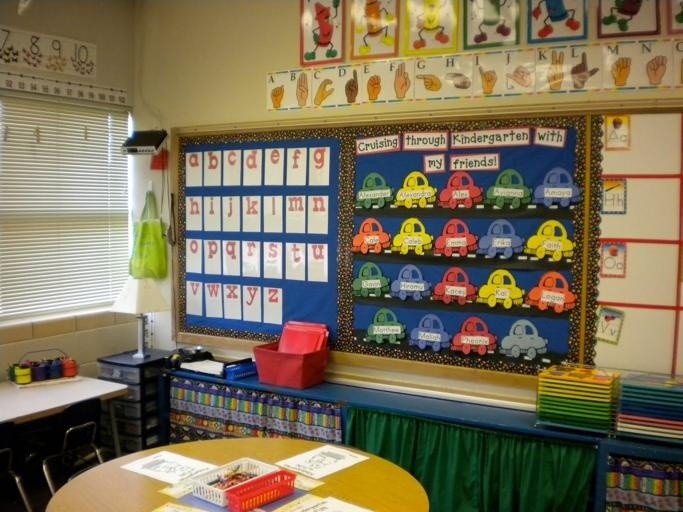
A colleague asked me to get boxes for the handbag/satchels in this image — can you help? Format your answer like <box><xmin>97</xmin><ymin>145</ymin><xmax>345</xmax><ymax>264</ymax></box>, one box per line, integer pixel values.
<box><xmin>128</xmin><ymin>189</ymin><xmax>169</xmax><ymax>282</ymax></box>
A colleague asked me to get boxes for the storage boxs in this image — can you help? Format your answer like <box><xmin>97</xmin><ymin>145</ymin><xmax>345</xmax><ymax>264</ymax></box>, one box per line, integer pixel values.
<box><xmin>254</xmin><ymin>341</ymin><xmax>329</xmax><ymax>390</ymax></box>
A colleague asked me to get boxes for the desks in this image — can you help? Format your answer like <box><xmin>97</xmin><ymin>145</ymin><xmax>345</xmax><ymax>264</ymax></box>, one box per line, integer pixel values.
<box><xmin>44</xmin><ymin>437</ymin><xmax>429</xmax><ymax>512</ymax></box>
<box><xmin>0</xmin><ymin>371</ymin><xmax>128</xmax><ymax>486</ymax></box>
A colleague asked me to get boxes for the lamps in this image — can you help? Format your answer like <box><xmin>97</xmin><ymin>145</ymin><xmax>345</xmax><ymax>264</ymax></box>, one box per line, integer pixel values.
<box><xmin>110</xmin><ymin>276</ymin><xmax>170</xmax><ymax>360</ymax></box>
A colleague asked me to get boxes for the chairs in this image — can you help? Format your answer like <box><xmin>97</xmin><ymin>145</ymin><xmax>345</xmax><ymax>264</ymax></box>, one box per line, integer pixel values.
<box><xmin>35</xmin><ymin>397</ymin><xmax>105</xmax><ymax>497</ymax></box>
<box><xmin>0</xmin><ymin>421</ymin><xmax>34</xmax><ymax>512</ymax></box>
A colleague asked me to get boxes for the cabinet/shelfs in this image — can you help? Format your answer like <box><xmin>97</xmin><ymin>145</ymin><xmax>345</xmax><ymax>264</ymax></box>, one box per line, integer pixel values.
<box><xmin>97</xmin><ymin>347</ymin><xmax>171</xmax><ymax>456</ymax></box>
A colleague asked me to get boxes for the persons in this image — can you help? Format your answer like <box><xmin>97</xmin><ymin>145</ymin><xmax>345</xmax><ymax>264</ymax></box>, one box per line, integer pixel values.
<box><xmin>647</xmin><ymin>55</ymin><xmax>668</xmax><ymax>84</ymax></box>
<box><xmin>272</xmin><ymin>85</ymin><xmax>284</xmax><ymax>109</ymax></box>
<box><xmin>611</xmin><ymin>56</ymin><xmax>632</xmax><ymax>87</ymax></box>
<box><xmin>546</xmin><ymin>50</ymin><xmax>564</xmax><ymax>90</ymax></box>
<box><xmin>314</xmin><ymin>79</ymin><xmax>334</xmax><ymax>105</ymax></box>
<box><xmin>416</xmin><ymin>74</ymin><xmax>441</xmax><ymax>92</ymax></box>
<box><xmin>367</xmin><ymin>76</ymin><xmax>382</xmax><ymax>100</ymax></box>
<box><xmin>394</xmin><ymin>63</ymin><xmax>411</xmax><ymax>98</ymax></box>
<box><xmin>444</xmin><ymin>72</ymin><xmax>471</xmax><ymax>90</ymax></box>
<box><xmin>296</xmin><ymin>73</ymin><xmax>309</xmax><ymax>106</ymax></box>
<box><xmin>479</xmin><ymin>67</ymin><xmax>497</xmax><ymax>93</ymax></box>
<box><xmin>345</xmin><ymin>70</ymin><xmax>358</xmax><ymax>104</ymax></box>
<box><xmin>570</xmin><ymin>52</ymin><xmax>599</xmax><ymax>89</ymax></box>
<box><xmin>506</xmin><ymin>65</ymin><xmax>533</xmax><ymax>88</ymax></box>
